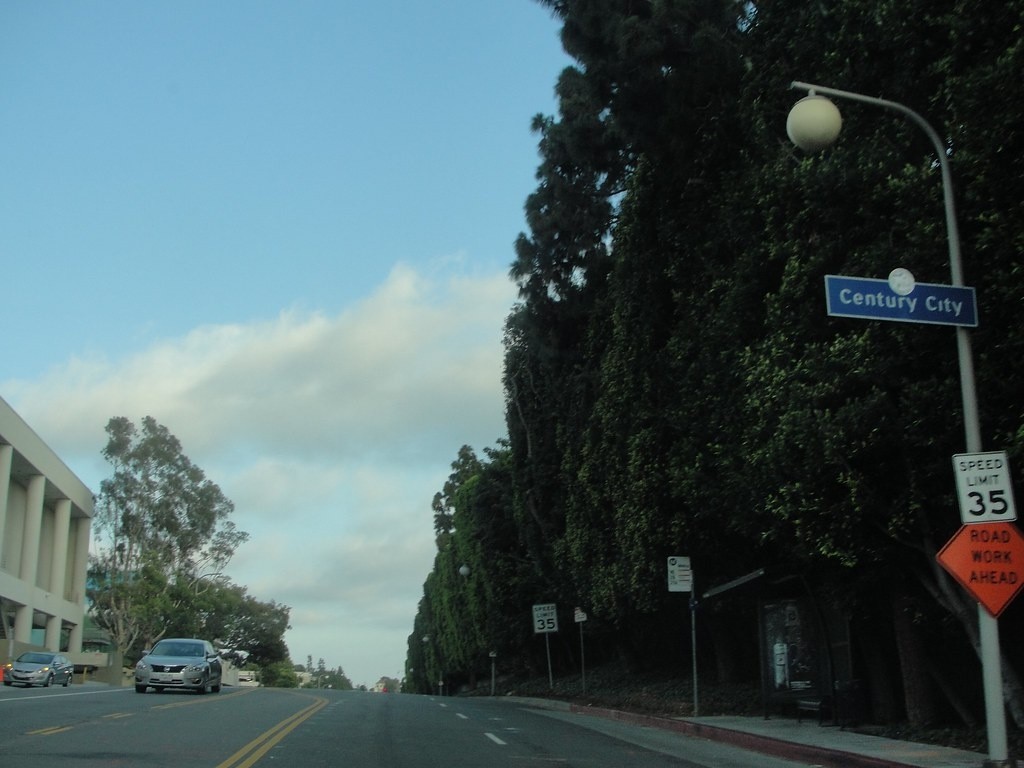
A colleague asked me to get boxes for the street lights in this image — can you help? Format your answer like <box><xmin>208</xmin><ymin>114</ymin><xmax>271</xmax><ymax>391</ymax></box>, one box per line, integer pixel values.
<box><xmin>786</xmin><ymin>78</ymin><xmax>1013</xmax><ymax>767</ymax></box>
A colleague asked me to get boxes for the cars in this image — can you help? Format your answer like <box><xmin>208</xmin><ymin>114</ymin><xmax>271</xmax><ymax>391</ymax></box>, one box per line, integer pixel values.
<box><xmin>5</xmin><ymin>651</ymin><xmax>75</xmax><ymax>688</ymax></box>
<box><xmin>133</xmin><ymin>639</ymin><xmax>224</xmax><ymax>695</ymax></box>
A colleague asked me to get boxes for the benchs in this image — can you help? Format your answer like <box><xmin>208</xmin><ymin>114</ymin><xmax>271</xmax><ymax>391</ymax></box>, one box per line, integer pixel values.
<box><xmin>797</xmin><ymin>699</ymin><xmax>833</xmax><ymax>726</ymax></box>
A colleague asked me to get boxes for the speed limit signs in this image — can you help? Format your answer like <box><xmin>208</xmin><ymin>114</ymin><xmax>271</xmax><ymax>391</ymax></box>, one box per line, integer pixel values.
<box><xmin>950</xmin><ymin>451</ymin><xmax>1019</xmax><ymax>525</ymax></box>
<box><xmin>533</xmin><ymin>603</ymin><xmax>558</xmax><ymax>633</ymax></box>
